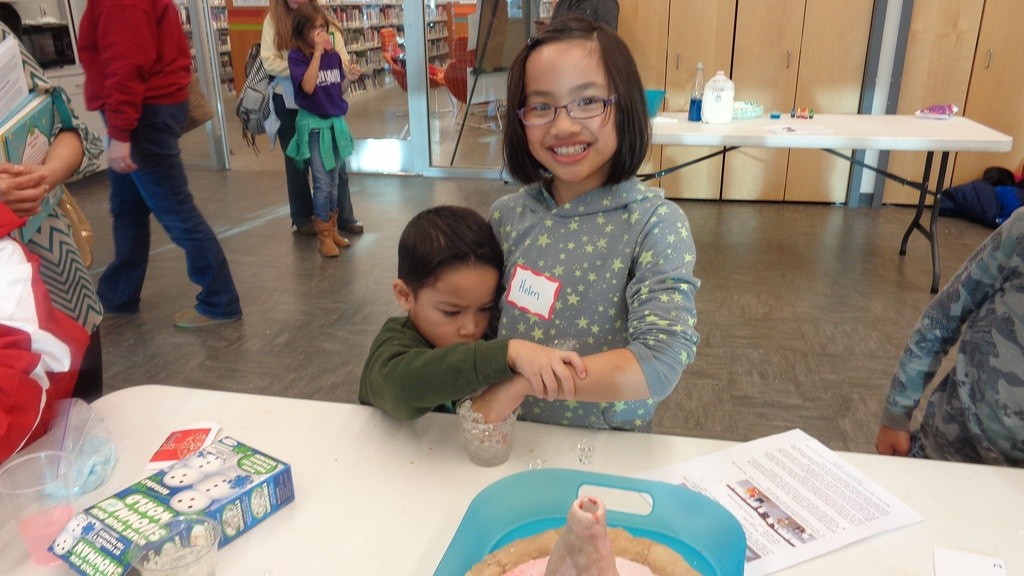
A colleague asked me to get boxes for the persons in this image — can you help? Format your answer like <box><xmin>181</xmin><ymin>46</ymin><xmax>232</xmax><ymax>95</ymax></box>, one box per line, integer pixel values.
<box><xmin>0</xmin><ymin>0</ymin><xmax>105</xmax><ymax>401</ymax></box>
<box><xmin>0</xmin><ymin>200</ymin><xmax>91</xmax><ymax>466</ymax></box>
<box><xmin>288</xmin><ymin>2</ymin><xmax>354</xmax><ymax>256</ymax></box>
<box><xmin>874</xmin><ymin>205</ymin><xmax>1024</xmax><ymax>468</ymax></box>
<box><xmin>76</xmin><ymin>0</ymin><xmax>245</xmax><ymax>329</ymax></box>
<box><xmin>550</xmin><ymin>0</ymin><xmax>619</xmax><ymax>32</ymax></box>
<box><xmin>261</xmin><ymin>0</ymin><xmax>364</xmax><ymax>235</ymax></box>
<box><xmin>470</xmin><ymin>16</ymin><xmax>701</xmax><ymax>432</ymax></box>
<box><xmin>359</xmin><ymin>204</ymin><xmax>588</xmax><ymax>423</ymax></box>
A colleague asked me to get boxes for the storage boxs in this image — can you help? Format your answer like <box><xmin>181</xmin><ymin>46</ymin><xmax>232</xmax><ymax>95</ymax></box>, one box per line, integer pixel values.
<box><xmin>46</xmin><ymin>436</ymin><xmax>297</xmax><ymax>575</ymax></box>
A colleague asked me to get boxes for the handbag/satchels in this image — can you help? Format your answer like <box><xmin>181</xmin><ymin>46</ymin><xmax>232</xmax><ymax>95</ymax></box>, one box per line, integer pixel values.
<box><xmin>175</xmin><ymin>69</ymin><xmax>215</xmax><ymax>134</ymax></box>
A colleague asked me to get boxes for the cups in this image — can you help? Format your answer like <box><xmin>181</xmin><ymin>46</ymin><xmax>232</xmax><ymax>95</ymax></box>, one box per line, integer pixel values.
<box><xmin>317</xmin><ymin>30</ymin><xmax>332</xmax><ymax>50</ymax></box>
<box><xmin>128</xmin><ymin>514</ymin><xmax>221</xmax><ymax>576</ymax></box>
<box><xmin>0</xmin><ymin>450</ymin><xmax>75</xmax><ymax>566</ymax></box>
<box><xmin>455</xmin><ymin>393</ymin><xmax>520</xmax><ymax>465</ymax></box>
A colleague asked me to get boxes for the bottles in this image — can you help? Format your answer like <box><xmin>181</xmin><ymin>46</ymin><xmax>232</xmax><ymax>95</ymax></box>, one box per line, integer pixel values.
<box><xmin>791</xmin><ymin>106</ymin><xmax>814</xmax><ymax>119</ymax></box>
<box><xmin>688</xmin><ymin>62</ymin><xmax>705</xmax><ymax>122</ymax></box>
<box><xmin>700</xmin><ymin>71</ymin><xmax>736</xmax><ymax>125</ymax></box>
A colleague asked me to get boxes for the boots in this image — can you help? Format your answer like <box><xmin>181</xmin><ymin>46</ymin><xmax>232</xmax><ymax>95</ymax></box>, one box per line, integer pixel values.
<box><xmin>332</xmin><ymin>216</ymin><xmax>350</xmax><ymax>247</ymax></box>
<box><xmin>315</xmin><ymin>218</ymin><xmax>339</xmax><ymax>259</ymax></box>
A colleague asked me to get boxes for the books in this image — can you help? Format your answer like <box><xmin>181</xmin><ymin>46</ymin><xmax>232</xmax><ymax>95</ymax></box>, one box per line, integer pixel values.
<box><xmin>324</xmin><ymin>6</ymin><xmax>448</xmax><ymax>98</ymax></box>
<box><xmin>0</xmin><ymin>91</ymin><xmax>64</xmax><ymax>246</ymax></box>
<box><xmin>176</xmin><ymin>9</ymin><xmax>235</xmax><ymax>98</ymax></box>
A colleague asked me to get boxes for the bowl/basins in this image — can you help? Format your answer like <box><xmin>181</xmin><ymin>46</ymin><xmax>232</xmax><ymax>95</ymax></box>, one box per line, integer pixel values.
<box><xmin>644</xmin><ymin>90</ymin><xmax>666</xmax><ymax>117</ymax></box>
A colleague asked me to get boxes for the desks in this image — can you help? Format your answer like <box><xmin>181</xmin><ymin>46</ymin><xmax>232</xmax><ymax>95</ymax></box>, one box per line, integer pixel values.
<box><xmin>0</xmin><ymin>384</ymin><xmax>1024</xmax><ymax>576</ymax></box>
<box><xmin>637</xmin><ymin>112</ymin><xmax>1013</xmax><ymax>294</ymax></box>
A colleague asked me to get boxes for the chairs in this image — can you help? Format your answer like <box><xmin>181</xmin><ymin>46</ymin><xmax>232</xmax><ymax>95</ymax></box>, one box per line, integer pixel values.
<box><xmin>381</xmin><ymin>28</ymin><xmax>502</xmax><ymax>132</ymax></box>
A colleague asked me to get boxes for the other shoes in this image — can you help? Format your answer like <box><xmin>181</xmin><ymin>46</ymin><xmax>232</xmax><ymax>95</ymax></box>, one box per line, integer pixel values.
<box><xmin>172</xmin><ymin>307</ymin><xmax>242</xmax><ymax>327</ymax></box>
<box><xmin>338</xmin><ymin>218</ymin><xmax>363</xmax><ymax>234</ymax></box>
<box><xmin>296</xmin><ymin>221</ymin><xmax>319</xmax><ymax>234</ymax></box>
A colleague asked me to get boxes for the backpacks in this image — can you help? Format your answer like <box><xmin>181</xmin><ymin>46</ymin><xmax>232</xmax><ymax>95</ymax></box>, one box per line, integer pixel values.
<box><xmin>235</xmin><ymin>42</ymin><xmax>274</xmax><ymax>136</ymax></box>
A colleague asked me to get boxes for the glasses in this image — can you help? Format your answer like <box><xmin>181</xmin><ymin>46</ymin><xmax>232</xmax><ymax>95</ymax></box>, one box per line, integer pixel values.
<box><xmin>516</xmin><ymin>92</ymin><xmax>621</xmax><ymax>127</ymax></box>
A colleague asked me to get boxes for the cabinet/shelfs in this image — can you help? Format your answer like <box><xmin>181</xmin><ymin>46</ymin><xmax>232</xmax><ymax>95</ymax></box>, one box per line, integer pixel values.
<box><xmin>175</xmin><ymin>0</ymin><xmax>235</xmax><ymax>100</ymax></box>
<box><xmin>225</xmin><ymin>0</ymin><xmax>453</xmax><ymax>99</ymax></box>
